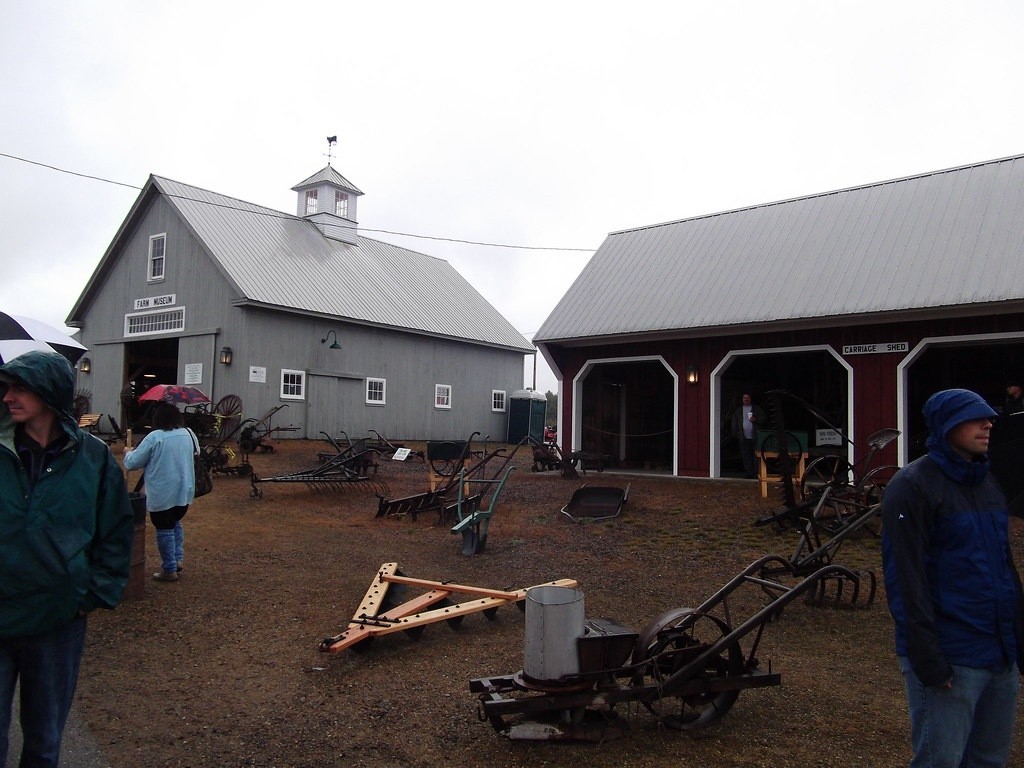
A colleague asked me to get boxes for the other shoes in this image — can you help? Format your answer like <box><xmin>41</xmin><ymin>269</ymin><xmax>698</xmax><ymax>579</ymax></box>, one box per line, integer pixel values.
<box><xmin>177</xmin><ymin>560</ymin><xmax>184</xmax><ymax>571</ymax></box>
<box><xmin>154</xmin><ymin>568</ymin><xmax>179</xmax><ymax>581</ymax></box>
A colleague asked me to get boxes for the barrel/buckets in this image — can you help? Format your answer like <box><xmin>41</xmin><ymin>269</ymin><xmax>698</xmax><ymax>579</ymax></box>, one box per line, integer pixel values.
<box><xmin>126</xmin><ymin>492</ymin><xmax>147</xmax><ymax>587</ymax></box>
<box><xmin>524</xmin><ymin>586</ymin><xmax>585</xmax><ymax>681</ymax></box>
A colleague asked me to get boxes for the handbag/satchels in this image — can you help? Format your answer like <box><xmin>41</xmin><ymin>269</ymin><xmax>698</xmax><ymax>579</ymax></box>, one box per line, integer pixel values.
<box><xmin>185</xmin><ymin>426</ymin><xmax>213</xmax><ymax>498</ymax></box>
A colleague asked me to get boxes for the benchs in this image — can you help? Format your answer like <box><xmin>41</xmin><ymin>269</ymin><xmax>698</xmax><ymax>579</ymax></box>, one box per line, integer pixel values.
<box><xmin>79</xmin><ymin>413</ymin><xmax>103</xmax><ymax>434</ymax></box>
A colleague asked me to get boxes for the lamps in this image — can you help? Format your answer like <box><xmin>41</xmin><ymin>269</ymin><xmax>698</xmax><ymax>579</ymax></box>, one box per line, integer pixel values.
<box><xmin>220</xmin><ymin>346</ymin><xmax>232</xmax><ymax>367</ymax></box>
<box><xmin>79</xmin><ymin>358</ymin><xmax>90</xmax><ymax>372</ymax></box>
<box><xmin>322</xmin><ymin>329</ymin><xmax>342</xmax><ymax>349</ymax></box>
<box><xmin>686</xmin><ymin>366</ymin><xmax>700</xmax><ymax>384</ymax></box>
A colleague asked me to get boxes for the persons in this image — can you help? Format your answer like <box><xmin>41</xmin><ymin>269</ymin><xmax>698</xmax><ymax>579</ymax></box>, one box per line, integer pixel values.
<box><xmin>121</xmin><ymin>371</ymin><xmax>157</xmax><ymax>433</ymax></box>
<box><xmin>995</xmin><ymin>375</ymin><xmax>1024</xmax><ymax>514</ymax></box>
<box><xmin>730</xmin><ymin>393</ymin><xmax>767</xmax><ymax>476</ymax></box>
<box><xmin>124</xmin><ymin>405</ymin><xmax>200</xmax><ymax>583</ymax></box>
<box><xmin>0</xmin><ymin>352</ymin><xmax>135</xmax><ymax>768</ymax></box>
<box><xmin>880</xmin><ymin>389</ymin><xmax>1024</xmax><ymax>768</ymax></box>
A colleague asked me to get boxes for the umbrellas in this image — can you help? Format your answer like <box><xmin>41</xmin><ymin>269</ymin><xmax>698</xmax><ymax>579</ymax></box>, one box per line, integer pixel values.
<box><xmin>138</xmin><ymin>384</ymin><xmax>215</xmax><ymax>406</ymax></box>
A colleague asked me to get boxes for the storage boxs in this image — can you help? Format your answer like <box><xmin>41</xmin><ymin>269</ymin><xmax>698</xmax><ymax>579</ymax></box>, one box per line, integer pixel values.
<box><xmin>757</xmin><ymin>430</ymin><xmax>807</xmax><ymax>449</ymax></box>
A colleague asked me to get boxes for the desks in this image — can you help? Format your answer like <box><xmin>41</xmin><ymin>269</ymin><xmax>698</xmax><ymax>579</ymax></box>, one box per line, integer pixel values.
<box><xmin>755</xmin><ymin>450</ymin><xmax>808</xmax><ymax>499</ymax></box>
<box><xmin>808</xmin><ymin>447</ymin><xmax>848</xmax><ymax>483</ymax></box>
